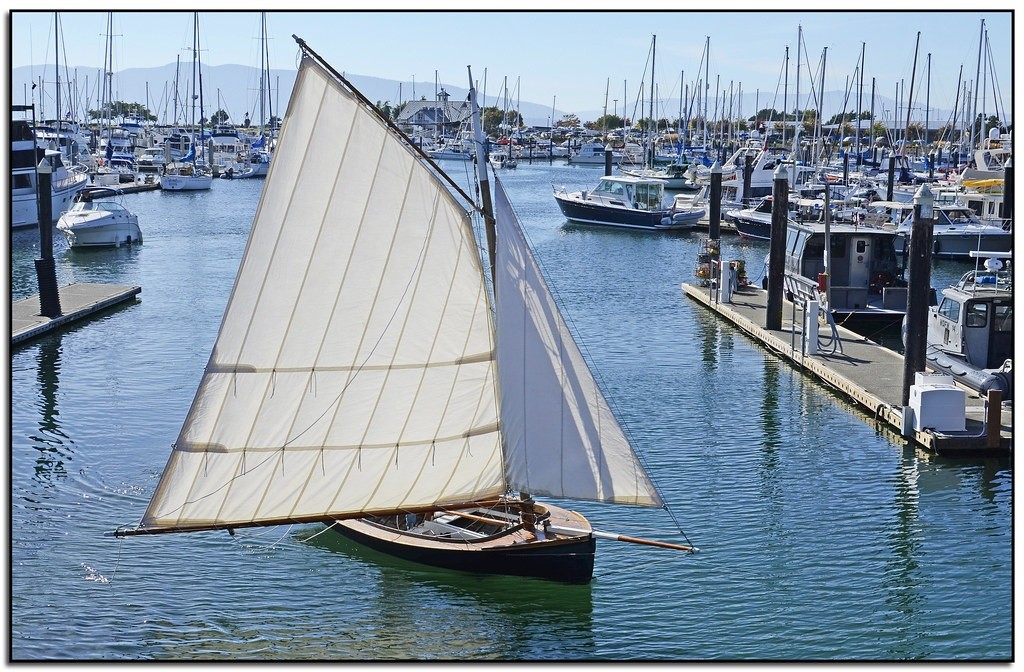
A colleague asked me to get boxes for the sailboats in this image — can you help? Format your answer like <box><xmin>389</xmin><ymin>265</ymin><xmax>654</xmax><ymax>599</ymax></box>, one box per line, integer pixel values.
<box><xmin>109</xmin><ymin>28</ymin><xmax>700</xmax><ymax>586</ymax></box>
<box><xmin>7</xmin><ymin>17</ymin><xmax>1017</xmax><ymax>395</ymax></box>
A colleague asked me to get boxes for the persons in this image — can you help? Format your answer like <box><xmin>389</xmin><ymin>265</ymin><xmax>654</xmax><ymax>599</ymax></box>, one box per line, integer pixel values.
<box><xmin>730</xmin><ymin>261</ymin><xmax>739</xmax><ymax>299</ymax></box>
